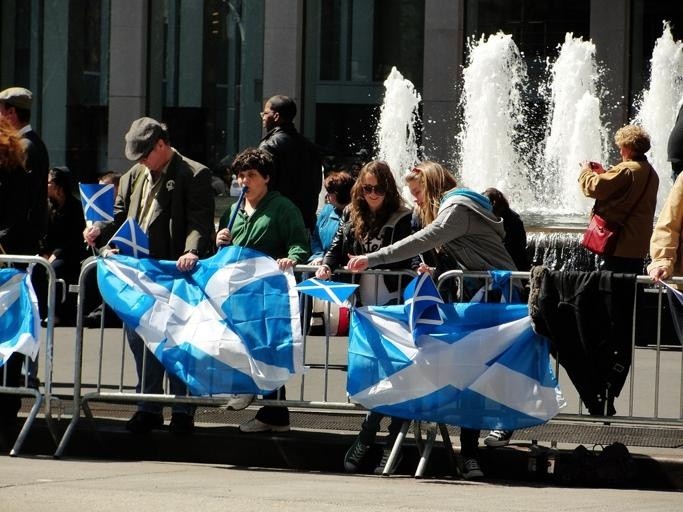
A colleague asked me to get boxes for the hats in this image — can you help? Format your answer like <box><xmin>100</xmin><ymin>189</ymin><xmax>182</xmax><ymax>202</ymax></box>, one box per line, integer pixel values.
<box><xmin>125</xmin><ymin>118</ymin><xmax>160</xmax><ymax>161</ymax></box>
<box><xmin>0</xmin><ymin>87</ymin><xmax>33</xmax><ymax>107</ymax></box>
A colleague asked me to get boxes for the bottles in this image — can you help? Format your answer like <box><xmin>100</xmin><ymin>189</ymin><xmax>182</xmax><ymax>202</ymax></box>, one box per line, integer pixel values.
<box><xmin>546</xmin><ymin>441</ymin><xmax>559</xmax><ymax>482</ymax></box>
<box><xmin>527</xmin><ymin>439</ymin><xmax>542</xmax><ymax>481</ymax></box>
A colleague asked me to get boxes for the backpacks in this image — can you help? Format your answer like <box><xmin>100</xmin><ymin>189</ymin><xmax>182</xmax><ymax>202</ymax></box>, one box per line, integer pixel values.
<box><xmin>579</xmin><ymin>214</ymin><xmax>613</xmax><ymax>254</ymax></box>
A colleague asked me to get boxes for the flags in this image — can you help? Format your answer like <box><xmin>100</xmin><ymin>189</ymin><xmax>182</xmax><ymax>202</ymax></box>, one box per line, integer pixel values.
<box><xmin>291</xmin><ymin>276</ymin><xmax>360</xmax><ymax>306</ymax></box>
<box><xmin>403</xmin><ymin>270</ymin><xmax>444</xmax><ymax>349</ymax></box>
<box><xmin>105</xmin><ymin>216</ymin><xmax>150</xmax><ymax>260</ymax></box>
<box><xmin>78</xmin><ymin>182</ymin><xmax>114</xmax><ymax>222</ymax></box>
<box><xmin>659</xmin><ymin>279</ymin><xmax>683</xmax><ymax>306</ymax></box>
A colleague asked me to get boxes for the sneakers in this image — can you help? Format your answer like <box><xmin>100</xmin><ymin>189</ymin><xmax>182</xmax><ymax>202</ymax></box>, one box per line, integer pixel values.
<box><xmin>239</xmin><ymin>416</ymin><xmax>289</xmax><ymax>434</ymax></box>
<box><xmin>456</xmin><ymin>454</ymin><xmax>483</xmax><ymax>479</ymax></box>
<box><xmin>125</xmin><ymin>412</ymin><xmax>163</xmax><ymax>434</ymax></box>
<box><xmin>226</xmin><ymin>393</ymin><xmax>257</xmax><ymax>411</ymax></box>
<box><xmin>345</xmin><ymin>438</ymin><xmax>370</xmax><ymax>471</ymax></box>
<box><xmin>374</xmin><ymin>448</ymin><xmax>403</xmax><ymax>475</ymax></box>
<box><xmin>485</xmin><ymin>422</ymin><xmax>512</xmax><ymax>446</ymax></box>
<box><xmin>169</xmin><ymin>413</ymin><xmax>194</xmax><ymax>438</ymax></box>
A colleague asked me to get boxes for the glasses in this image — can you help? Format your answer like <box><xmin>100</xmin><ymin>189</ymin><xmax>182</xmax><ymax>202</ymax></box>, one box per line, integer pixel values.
<box><xmin>363</xmin><ymin>184</ymin><xmax>385</xmax><ymax>196</ymax></box>
<box><xmin>260</xmin><ymin>111</ymin><xmax>273</xmax><ymax>117</ymax></box>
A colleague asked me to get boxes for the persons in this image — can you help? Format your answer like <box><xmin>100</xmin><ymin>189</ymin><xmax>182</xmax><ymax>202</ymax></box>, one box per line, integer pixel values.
<box><xmin>307</xmin><ymin>171</ymin><xmax>355</xmax><ymax>266</ymax></box>
<box><xmin>83</xmin><ymin>117</ymin><xmax>216</xmax><ymax>439</ymax></box>
<box><xmin>480</xmin><ymin>187</ymin><xmax>526</xmax><ymax>272</ymax></box>
<box><xmin>96</xmin><ymin>170</ymin><xmax>122</xmax><ymax>202</ymax></box>
<box><xmin>216</xmin><ymin>148</ymin><xmax>310</xmax><ymax>433</ymax></box>
<box><xmin>257</xmin><ymin>95</ymin><xmax>310</xmax><ymax>205</ymax></box>
<box><xmin>0</xmin><ymin>86</ymin><xmax>49</xmax><ymax>247</ymax></box>
<box><xmin>577</xmin><ymin>125</ymin><xmax>659</xmax><ymax>274</ymax></box>
<box><xmin>646</xmin><ymin>170</ymin><xmax>683</xmax><ymax>282</ymax></box>
<box><xmin>315</xmin><ymin>161</ymin><xmax>436</xmax><ymax>476</ymax></box>
<box><xmin>0</xmin><ymin>115</ymin><xmax>38</xmax><ymax>269</ymax></box>
<box><xmin>40</xmin><ymin>164</ymin><xmax>81</xmax><ymax>327</ymax></box>
<box><xmin>343</xmin><ymin>160</ymin><xmax>526</xmax><ymax>478</ymax></box>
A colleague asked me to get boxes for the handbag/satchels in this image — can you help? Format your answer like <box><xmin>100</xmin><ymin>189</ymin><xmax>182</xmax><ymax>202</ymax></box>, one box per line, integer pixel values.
<box><xmin>579</xmin><ymin>165</ymin><xmax>653</xmax><ymax>257</ymax></box>
